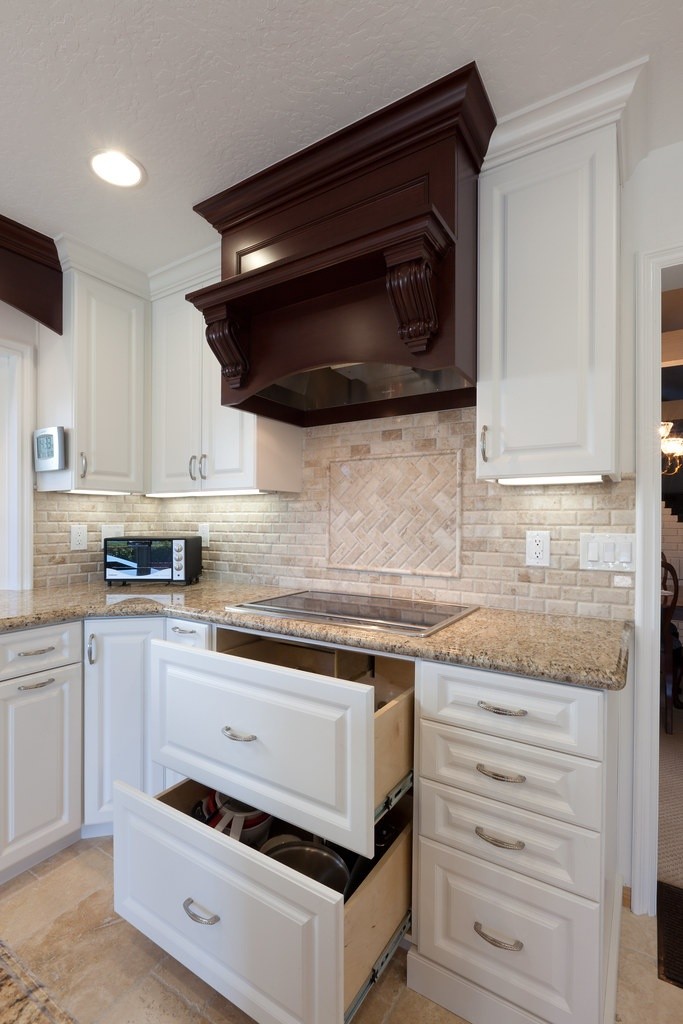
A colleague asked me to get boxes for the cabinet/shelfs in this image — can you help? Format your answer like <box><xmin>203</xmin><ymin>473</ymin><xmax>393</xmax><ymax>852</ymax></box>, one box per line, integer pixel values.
<box><xmin>406</xmin><ymin>657</ymin><xmax>623</xmax><ymax>1024</ymax></box>
<box><xmin>474</xmin><ymin>123</ymin><xmax>634</xmax><ymax>488</ymax></box>
<box><xmin>81</xmin><ymin>616</ymin><xmax>148</xmax><ymax>840</ymax></box>
<box><xmin>34</xmin><ymin>232</ymin><xmax>145</xmax><ymax>497</ymax></box>
<box><xmin>108</xmin><ymin>637</ymin><xmax>414</xmax><ymax>1024</ymax></box>
<box><xmin>0</xmin><ymin>619</ymin><xmax>83</xmax><ymax>885</ymax></box>
<box><xmin>147</xmin><ymin>241</ymin><xmax>304</xmax><ymax>498</ymax></box>
<box><xmin>163</xmin><ymin>616</ymin><xmax>213</xmax><ymax>791</ymax></box>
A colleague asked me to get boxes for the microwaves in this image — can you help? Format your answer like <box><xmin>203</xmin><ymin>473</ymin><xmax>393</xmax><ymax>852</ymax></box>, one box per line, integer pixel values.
<box><xmin>104</xmin><ymin>536</ymin><xmax>202</xmax><ymax>587</ymax></box>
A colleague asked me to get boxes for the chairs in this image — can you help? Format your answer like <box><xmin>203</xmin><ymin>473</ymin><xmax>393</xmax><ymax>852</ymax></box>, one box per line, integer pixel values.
<box><xmin>661</xmin><ymin>561</ymin><xmax>679</xmax><ymax>734</ymax></box>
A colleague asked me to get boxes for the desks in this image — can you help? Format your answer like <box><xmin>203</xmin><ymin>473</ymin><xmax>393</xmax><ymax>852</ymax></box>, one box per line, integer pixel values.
<box><xmin>662</xmin><ymin>585</ymin><xmax>683</xmax><ymax>710</ymax></box>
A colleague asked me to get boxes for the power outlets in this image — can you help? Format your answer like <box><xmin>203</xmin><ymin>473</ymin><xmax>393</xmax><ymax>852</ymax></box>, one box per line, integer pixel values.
<box><xmin>70</xmin><ymin>524</ymin><xmax>88</xmax><ymax>550</ymax></box>
<box><xmin>198</xmin><ymin>523</ymin><xmax>210</xmax><ymax>548</ymax></box>
<box><xmin>525</xmin><ymin>530</ymin><xmax>551</xmax><ymax>567</ymax></box>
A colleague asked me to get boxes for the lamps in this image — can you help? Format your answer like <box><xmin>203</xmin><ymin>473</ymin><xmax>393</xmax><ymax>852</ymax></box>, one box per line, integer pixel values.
<box><xmin>661</xmin><ymin>421</ymin><xmax>683</xmax><ymax>475</ymax></box>
